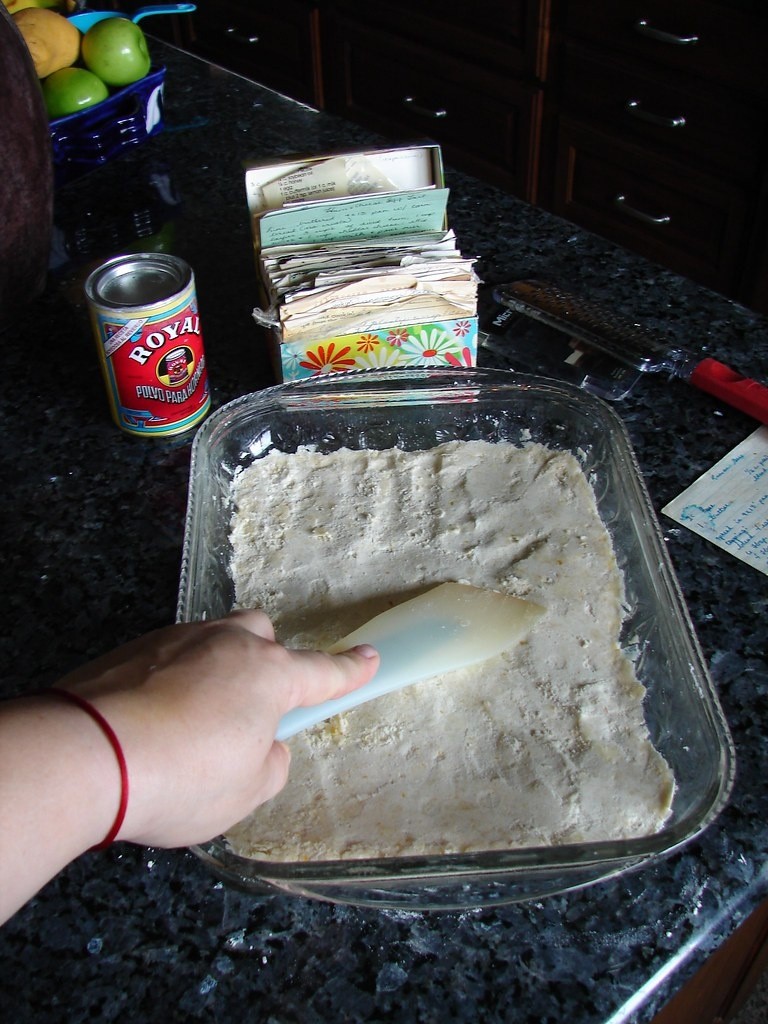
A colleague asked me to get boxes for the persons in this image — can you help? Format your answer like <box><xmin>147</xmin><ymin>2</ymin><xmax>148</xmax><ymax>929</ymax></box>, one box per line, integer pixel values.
<box><xmin>0</xmin><ymin>607</ymin><xmax>378</xmax><ymax>927</ymax></box>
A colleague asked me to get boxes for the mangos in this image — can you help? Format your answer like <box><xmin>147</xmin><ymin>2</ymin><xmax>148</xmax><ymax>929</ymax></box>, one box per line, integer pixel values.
<box><xmin>11</xmin><ymin>7</ymin><xmax>81</xmax><ymax>78</ymax></box>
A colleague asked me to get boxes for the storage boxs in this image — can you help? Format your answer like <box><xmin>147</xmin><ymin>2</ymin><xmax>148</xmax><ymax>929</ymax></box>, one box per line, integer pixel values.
<box><xmin>49</xmin><ymin>62</ymin><xmax>168</xmax><ymax>180</ymax></box>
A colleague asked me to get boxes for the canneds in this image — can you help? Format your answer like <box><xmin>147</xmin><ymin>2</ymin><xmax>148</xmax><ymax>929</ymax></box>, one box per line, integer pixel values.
<box><xmin>84</xmin><ymin>252</ymin><xmax>211</xmax><ymax>438</ymax></box>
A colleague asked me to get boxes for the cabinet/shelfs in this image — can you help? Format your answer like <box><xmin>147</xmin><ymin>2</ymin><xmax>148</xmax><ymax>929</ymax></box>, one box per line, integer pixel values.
<box><xmin>89</xmin><ymin>0</ymin><xmax>767</xmax><ymax>310</ymax></box>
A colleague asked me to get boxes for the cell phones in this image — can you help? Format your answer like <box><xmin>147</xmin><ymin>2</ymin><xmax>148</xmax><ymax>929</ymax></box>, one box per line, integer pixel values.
<box><xmin>476</xmin><ymin>299</ymin><xmax>645</xmax><ymax>401</ymax></box>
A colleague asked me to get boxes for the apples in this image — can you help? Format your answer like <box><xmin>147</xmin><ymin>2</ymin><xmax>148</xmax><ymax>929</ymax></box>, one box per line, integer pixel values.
<box><xmin>39</xmin><ymin>16</ymin><xmax>151</xmax><ymax>121</ymax></box>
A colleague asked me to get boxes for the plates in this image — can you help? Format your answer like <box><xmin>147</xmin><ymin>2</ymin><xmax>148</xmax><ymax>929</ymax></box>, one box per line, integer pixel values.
<box><xmin>172</xmin><ymin>364</ymin><xmax>737</xmax><ymax>913</ymax></box>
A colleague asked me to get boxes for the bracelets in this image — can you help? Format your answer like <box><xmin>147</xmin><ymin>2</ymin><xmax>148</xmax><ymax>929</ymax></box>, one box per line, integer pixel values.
<box><xmin>16</xmin><ymin>684</ymin><xmax>126</xmax><ymax>850</ymax></box>
